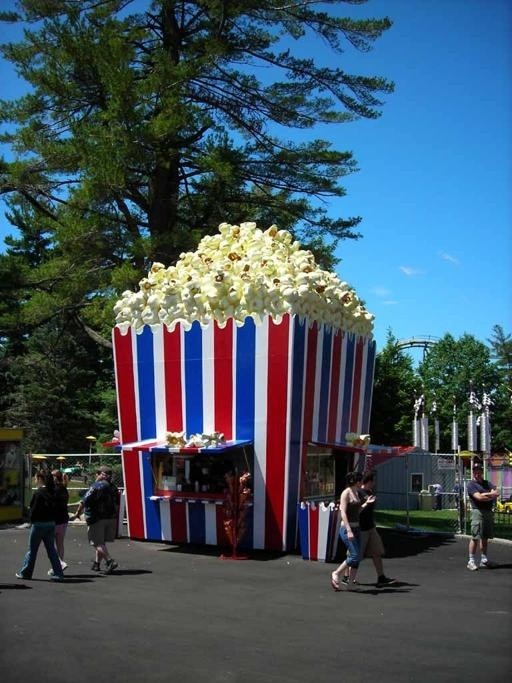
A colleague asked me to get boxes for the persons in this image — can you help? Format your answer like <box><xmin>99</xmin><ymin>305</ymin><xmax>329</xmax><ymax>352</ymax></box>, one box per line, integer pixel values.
<box><xmin>342</xmin><ymin>473</ymin><xmax>397</xmax><ymax>589</ymax></box>
<box><xmin>332</xmin><ymin>471</ymin><xmax>377</xmax><ymax>590</ymax></box>
<box><xmin>48</xmin><ymin>471</ymin><xmax>69</xmax><ymax>576</ymax></box>
<box><xmin>71</xmin><ymin>465</ymin><xmax>120</xmax><ymax>572</ymax></box>
<box><xmin>431</xmin><ymin>481</ymin><xmax>443</xmax><ymax>511</ymax></box>
<box><xmin>467</xmin><ymin>462</ymin><xmax>501</xmax><ymax>571</ymax></box>
<box><xmin>453</xmin><ymin>478</ymin><xmax>463</xmax><ymax>510</ymax></box>
<box><xmin>16</xmin><ymin>470</ymin><xmax>65</xmax><ymax>581</ymax></box>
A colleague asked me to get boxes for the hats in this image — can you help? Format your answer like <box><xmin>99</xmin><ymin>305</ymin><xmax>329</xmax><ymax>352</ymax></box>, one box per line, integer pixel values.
<box><xmin>472</xmin><ymin>463</ymin><xmax>481</xmax><ymax>470</ymax></box>
<box><xmin>96</xmin><ymin>466</ymin><xmax>112</xmax><ymax>475</ymax></box>
<box><xmin>51</xmin><ymin>470</ymin><xmax>62</xmax><ymax>477</ymax></box>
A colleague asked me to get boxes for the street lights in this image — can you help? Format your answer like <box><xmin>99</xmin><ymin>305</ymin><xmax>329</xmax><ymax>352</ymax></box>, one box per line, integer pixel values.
<box><xmin>56</xmin><ymin>456</ymin><xmax>66</xmax><ymax>468</ymax></box>
<box><xmin>32</xmin><ymin>455</ymin><xmax>47</xmax><ymax>472</ymax></box>
<box><xmin>85</xmin><ymin>434</ymin><xmax>96</xmax><ymax>467</ymax></box>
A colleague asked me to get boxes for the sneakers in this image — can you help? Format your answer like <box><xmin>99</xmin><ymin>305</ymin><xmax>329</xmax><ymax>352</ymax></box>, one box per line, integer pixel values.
<box><xmin>15</xmin><ymin>559</ymin><xmax>118</xmax><ymax>581</ymax></box>
<box><xmin>331</xmin><ymin>571</ymin><xmax>397</xmax><ymax>592</ymax></box>
<box><xmin>467</xmin><ymin>556</ymin><xmax>488</xmax><ymax>570</ymax></box>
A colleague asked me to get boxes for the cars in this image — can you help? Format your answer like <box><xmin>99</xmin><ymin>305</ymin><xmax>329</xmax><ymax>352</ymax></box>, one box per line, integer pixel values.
<box><xmin>492</xmin><ymin>497</ymin><xmax>512</xmax><ymax>513</ymax></box>
